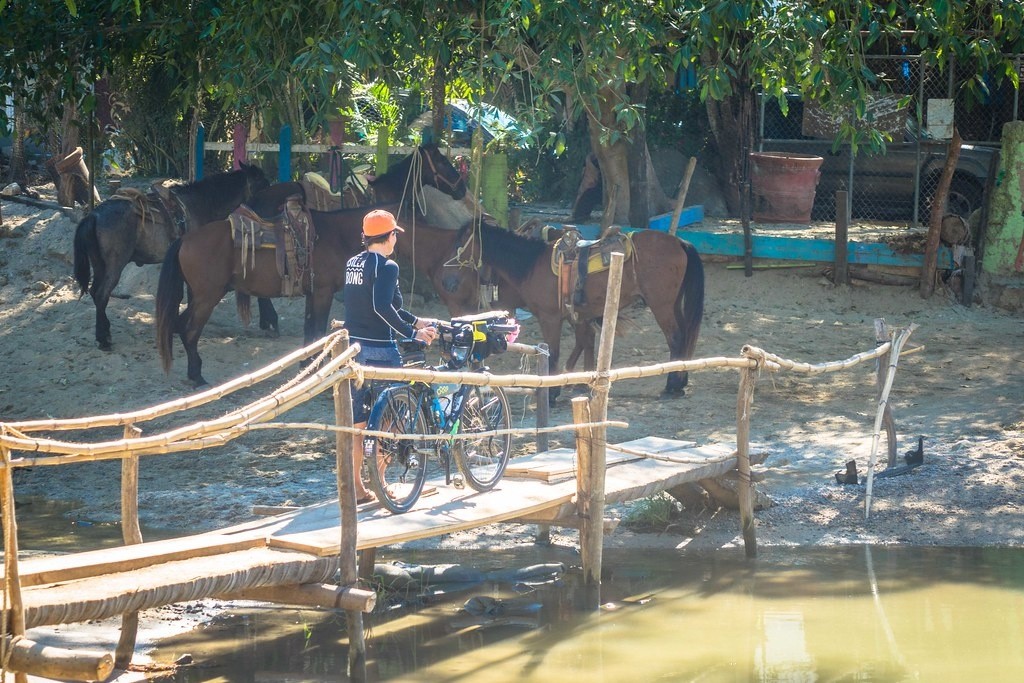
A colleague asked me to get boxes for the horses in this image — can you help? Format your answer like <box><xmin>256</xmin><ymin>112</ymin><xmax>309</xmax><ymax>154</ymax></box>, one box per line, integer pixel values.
<box><xmin>156</xmin><ymin>138</ymin><xmax>463</xmax><ymax>393</ymax></box>
<box><xmin>73</xmin><ymin>158</ymin><xmax>271</xmax><ymax>351</ymax></box>
<box><xmin>428</xmin><ymin>216</ymin><xmax>705</xmax><ymax>397</ymax></box>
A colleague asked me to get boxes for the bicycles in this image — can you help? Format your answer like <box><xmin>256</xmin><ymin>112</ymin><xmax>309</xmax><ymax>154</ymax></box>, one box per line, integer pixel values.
<box><xmin>364</xmin><ymin>310</ymin><xmax>515</xmax><ymax>514</ymax></box>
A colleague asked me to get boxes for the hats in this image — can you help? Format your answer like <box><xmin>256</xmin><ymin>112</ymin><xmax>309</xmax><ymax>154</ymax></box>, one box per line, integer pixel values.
<box><xmin>363</xmin><ymin>209</ymin><xmax>405</xmax><ymax>236</ymax></box>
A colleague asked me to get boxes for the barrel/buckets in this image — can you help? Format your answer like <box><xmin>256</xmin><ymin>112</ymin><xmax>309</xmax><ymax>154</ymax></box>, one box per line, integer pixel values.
<box><xmin>749</xmin><ymin>152</ymin><xmax>824</xmax><ymax>225</ymax></box>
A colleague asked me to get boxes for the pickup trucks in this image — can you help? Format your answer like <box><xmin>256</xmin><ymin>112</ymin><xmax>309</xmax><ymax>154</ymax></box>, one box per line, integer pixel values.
<box><xmin>749</xmin><ymin>82</ymin><xmax>1000</xmax><ymax>223</ymax></box>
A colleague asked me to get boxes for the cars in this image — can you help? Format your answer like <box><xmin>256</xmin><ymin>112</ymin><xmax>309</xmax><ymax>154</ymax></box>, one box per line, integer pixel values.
<box><xmin>384</xmin><ymin>92</ymin><xmax>542</xmax><ymax>155</ymax></box>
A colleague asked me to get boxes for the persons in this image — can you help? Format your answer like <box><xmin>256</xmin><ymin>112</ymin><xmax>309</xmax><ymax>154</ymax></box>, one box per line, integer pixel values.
<box><xmin>343</xmin><ymin>210</ymin><xmax>436</xmax><ymax>504</ymax></box>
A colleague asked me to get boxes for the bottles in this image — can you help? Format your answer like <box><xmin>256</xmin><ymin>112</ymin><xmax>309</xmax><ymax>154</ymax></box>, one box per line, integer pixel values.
<box><xmin>431</xmin><ymin>395</ymin><xmax>445</xmax><ymax>427</ymax></box>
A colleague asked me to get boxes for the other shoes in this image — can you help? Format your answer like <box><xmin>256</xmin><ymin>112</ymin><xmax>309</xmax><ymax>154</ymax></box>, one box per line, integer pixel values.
<box><xmin>387</xmin><ymin>490</ymin><xmax>396</xmax><ymax>499</ymax></box>
<box><xmin>357</xmin><ymin>489</ymin><xmax>375</xmax><ymax>504</ymax></box>
